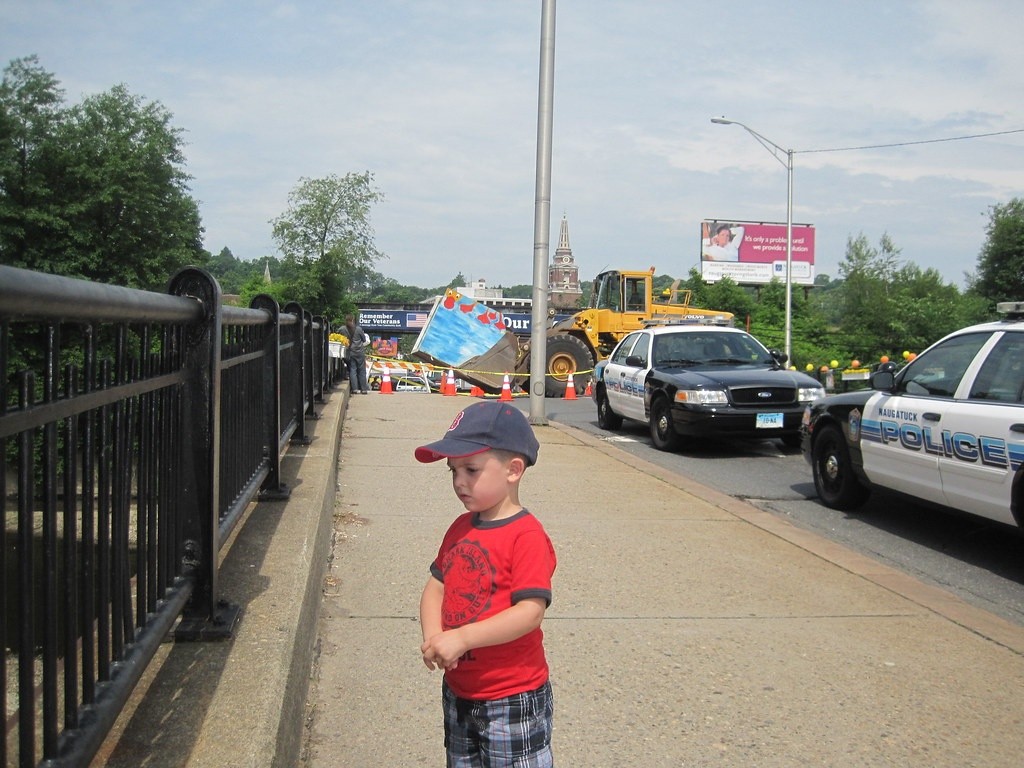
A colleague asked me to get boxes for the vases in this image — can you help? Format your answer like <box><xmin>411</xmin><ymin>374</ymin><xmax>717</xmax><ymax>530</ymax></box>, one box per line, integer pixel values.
<box><xmin>329</xmin><ymin>342</ymin><xmax>346</xmax><ymax>359</ymax></box>
<box><xmin>841</xmin><ymin>373</ymin><xmax>870</xmax><ymax>380</ymax></box>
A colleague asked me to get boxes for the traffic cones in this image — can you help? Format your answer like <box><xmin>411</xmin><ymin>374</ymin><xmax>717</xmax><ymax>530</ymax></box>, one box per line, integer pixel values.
<box><xmin>584</xmin><ymin>377</ymin><xmax>593</xmax><ymax>397</ymax></box>
<box><xmin>470</xmin><ymin>384</ymin><xmax>484</xmax><ymax>397</ymax></box>
<box><xmin>443</xmin><ymin>365</ymin><xmax>458</xmax><ymax>396</ymax></box>
<box><xmin>439</xmin><ymin>373</ymin><xmax>447</xmax><ymax>394</ymax></box>
<box><xmin>379</xmin><ymin>365</ymin><xmax>394</xmax><ymax>394</ymax></box>
<box><xmin>562</xmin><ymin>370</ymin><xmax>579</xmax><ymax>400</ymax></box>
<box><xmin>498</xmin><ymin>370</ymin><xmax>515</xmax><ymax>401</ymax></box>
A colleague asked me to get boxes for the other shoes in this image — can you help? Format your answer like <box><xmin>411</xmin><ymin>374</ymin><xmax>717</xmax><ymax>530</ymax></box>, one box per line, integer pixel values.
<box><xmin>352</xmin><ymin>390</ymin><xmax>368</xmax><ymax>394</ymax></box>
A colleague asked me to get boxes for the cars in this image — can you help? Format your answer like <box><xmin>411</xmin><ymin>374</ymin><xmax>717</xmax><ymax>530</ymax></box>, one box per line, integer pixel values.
<box><xmin>591</xmin><ymin>319</ymin><xmax>827</xmax><ymax>451</ymax></box>
<box><xmin>800</xmin><ymin>301</ymin><xmax>1023</xmax><ymax>528</ymax></box>
<box><xmin>397</xmin><ymin>377</ymin><xmax>431</xmax><ymax>392</ymax></box>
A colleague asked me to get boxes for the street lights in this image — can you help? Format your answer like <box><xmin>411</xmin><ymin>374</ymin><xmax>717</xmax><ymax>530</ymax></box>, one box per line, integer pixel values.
<box><xmin>711</xmin><ymin>117</ymin><xmax>795</xmax><ymax>370</ymax></box>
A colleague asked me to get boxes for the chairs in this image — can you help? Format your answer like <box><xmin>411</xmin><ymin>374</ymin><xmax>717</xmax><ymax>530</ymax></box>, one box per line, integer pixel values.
<box><xmin>705</xmin><ymin>342</ymin><xmax>728</xmax><ymax>358</ymax></box>
<box><xmin>629</xmin><ymin>291</ymin><xmax>644</xmax><ymax>311</ymax></box>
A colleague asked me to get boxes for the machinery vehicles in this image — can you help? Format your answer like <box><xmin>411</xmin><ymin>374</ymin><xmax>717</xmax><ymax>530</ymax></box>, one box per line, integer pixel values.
<box><xmin>444</xmin><ymin>266</ymin><xmax>734</xmax><ymax>398</ymax></box>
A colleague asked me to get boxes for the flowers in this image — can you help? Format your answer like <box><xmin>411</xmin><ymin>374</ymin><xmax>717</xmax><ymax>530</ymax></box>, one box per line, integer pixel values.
<box><xmin>329</xmin><ymin>333</ymin><xmax>350</xmax><ymax>347</ymax></box>
<box><xmin>845</xmin><ymin>368</ymin><xmax>869</xmax><ymax>375</ymax></box>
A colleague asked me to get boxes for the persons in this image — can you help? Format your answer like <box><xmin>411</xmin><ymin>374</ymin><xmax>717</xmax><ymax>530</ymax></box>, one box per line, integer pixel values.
<box><xmin>414</xmin><ymin>402</ymin><xmax>556</xmax><ymax>768</ymax></box>
<box><xmin>336</xmin><ymin>314</ymin><xmax>371</xmax><ymax>395</ymax></box>
<box><xmin>702</xmin><ymin>225</ymin><xmax>745</xmax><ymax>262</ymax></box>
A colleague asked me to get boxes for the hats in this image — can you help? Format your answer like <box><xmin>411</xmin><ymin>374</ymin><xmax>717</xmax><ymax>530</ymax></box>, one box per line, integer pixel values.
<box><xmin>414</xmin><ymin>402</ymin><xmax>541</xmax><ymax>467</ymax></box>
